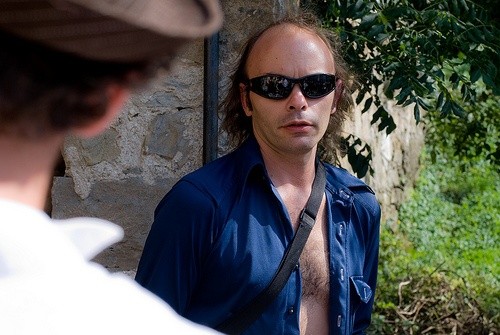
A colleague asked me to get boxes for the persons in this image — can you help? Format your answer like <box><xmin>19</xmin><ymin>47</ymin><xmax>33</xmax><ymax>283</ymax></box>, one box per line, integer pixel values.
<box><xmin>135</xmin><ymin>15</ymin><xmax>382</xmax><ymax>335</ymax></box>
<box><xmin>0</xmin><ymin>0</ymin><xmax>225</xmax><ymax>334</ymax></box>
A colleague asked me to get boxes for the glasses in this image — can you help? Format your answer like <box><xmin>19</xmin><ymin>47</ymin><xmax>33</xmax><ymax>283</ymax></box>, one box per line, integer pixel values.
<box><xmin>249</xmin><ymin>73</ymin><xmax>336</xmax><ymax>99</ymax></box>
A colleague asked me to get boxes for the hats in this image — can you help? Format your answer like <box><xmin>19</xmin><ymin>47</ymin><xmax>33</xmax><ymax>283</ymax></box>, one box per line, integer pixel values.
<box><xmin>0</xmin><ymin>0</ymin><xmax>223</xmax><ymax>63</ymax></box>
